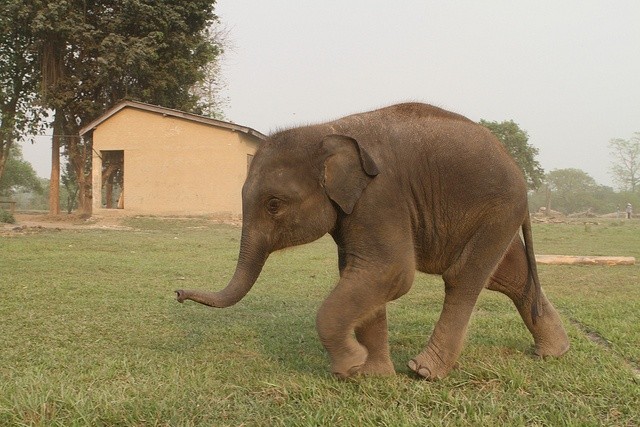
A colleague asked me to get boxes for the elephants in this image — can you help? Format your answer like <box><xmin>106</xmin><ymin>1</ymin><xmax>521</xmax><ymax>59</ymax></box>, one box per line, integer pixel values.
<box><xmin>175</xmin><ymin>100</ymin><xmax>570</xmax><ymax>382</ymax></box>
